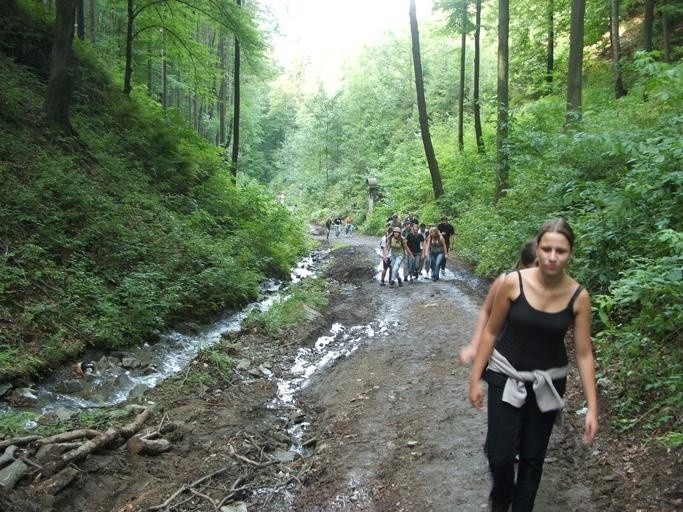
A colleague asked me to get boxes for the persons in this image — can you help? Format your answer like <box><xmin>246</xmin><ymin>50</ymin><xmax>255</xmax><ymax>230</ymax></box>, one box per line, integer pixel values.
<box><xmin>326</xmin><ymin>219</ymin><xmax>332</xmax><ymax>239</ymax></box>
<box><xmin>380</xmin><ymin>212</ymin><xmax>455</xmax><ymax>288</ymax></box>
<box><xmin>468</xmin><ymin>219</ymin><xmax>599</xmax><ymax>512</ymax></box>
<box><xmin>461</xmin><ymin>240</ymin><xmax>537</xmax><ymax>365</ymax></box>
<box><xmin>335</xmin><ymin>217</ymin><xmax>342</xmax><ymax>238</ymax></box>
<box><xmin>345</xmin><ymin>216</ymin><xmax>352</xmax><ymax>237</ymax></box>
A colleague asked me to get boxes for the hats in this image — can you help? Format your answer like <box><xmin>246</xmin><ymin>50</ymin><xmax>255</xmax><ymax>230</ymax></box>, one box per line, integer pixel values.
<box><xmin>393</xmin><ymin>227</ymin><xmax>401</xmax><ymax>233</ymax></box>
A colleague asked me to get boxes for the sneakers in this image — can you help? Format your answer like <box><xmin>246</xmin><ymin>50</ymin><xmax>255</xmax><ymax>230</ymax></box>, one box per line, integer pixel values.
<box><xmin>380</xmin><ymin>269</ymin><xmax>445</xmax><ymax>288</ymax></box>
